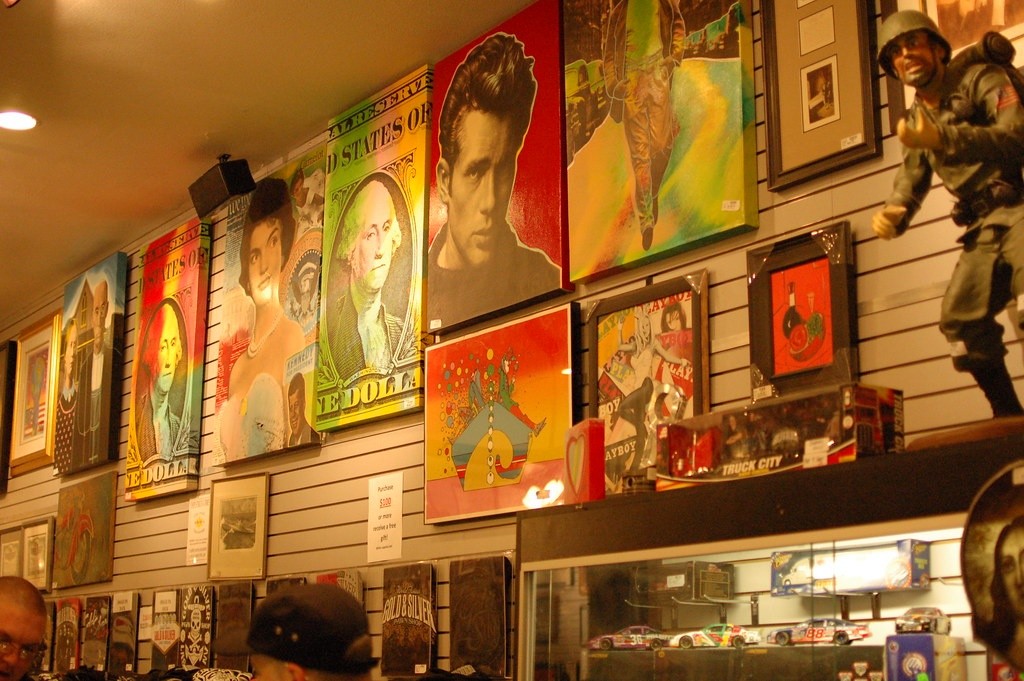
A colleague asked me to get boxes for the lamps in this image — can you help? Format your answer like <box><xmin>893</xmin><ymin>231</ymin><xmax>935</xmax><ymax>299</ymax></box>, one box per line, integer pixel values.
<box><xmin>187</xmin><ymin>153</ymin><xmax>258</xmax><ymax>220</ymax></box>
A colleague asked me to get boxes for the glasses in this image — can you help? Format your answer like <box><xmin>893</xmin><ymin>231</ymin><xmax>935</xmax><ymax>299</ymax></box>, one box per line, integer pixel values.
<box><xmin>0</xmin><ymin>640</ymin><xmax>40</xmax><ymax>661</ymax></box>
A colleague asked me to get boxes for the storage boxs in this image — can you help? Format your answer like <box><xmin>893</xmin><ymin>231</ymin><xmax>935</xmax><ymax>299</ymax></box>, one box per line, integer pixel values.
<box><xmin>770</xmin><ymin>539</ymin><xmax>931</xmax><ymax>597</ymax></box>
<box><xmin>655</xmin><ymin>383</ymin><xmax>904</xmax><ymax>493</ymax></box>
<box><xmin>627</xmin><ymin>561</ymin><xmax>735</xmax><ymax>605</ymax></box>
<box><xmin>883</xmin><ymin>634</ymin><xmax>967</xmax><ymax>681</ymax></box>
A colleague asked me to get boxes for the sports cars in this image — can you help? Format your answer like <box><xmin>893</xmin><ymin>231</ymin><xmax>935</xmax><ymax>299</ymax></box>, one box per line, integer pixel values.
<box><xmin>893</xmin><ymin>607</ymin><xmax>952</xmax><ymax>634</ymax></box>
<box><xmin>766</xmin><ymin>617</ymin><xmax>870</xmax><ymax>647</ymax></box>
<box><xmin>668</xmin><ymin>623</ymin><xmax>761</xmax><ymax>650</ymax></box>
<box><xmin>588</xmin><ymin>624</ymin><xmax>673</xmax><ymax>651</ymax></box>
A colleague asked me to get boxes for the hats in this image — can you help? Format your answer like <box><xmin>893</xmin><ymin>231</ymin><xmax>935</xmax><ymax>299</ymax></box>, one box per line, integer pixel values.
<box><xmin>214</xmin><ymin>583</ymin><xmax>381</xmax><ymax>676</ymax></box>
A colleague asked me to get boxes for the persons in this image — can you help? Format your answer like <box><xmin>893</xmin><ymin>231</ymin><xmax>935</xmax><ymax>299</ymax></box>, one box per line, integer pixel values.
<box><xmin>245</xmin><ymin>583</ymin><xmax>382</xmax><ymax>681</ymax></box>
<box><xmin>871</xmin><ymin>9</ymin><xmax>1024</xmax><ymax>421</ymax></box>
<box><xmin>0</xmin><ymin>576</ymin><xmax>47</xmax><ymax>681</ymax></box>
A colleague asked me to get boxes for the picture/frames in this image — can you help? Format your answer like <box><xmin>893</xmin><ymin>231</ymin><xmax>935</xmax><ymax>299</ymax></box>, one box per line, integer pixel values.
<box><xmin>20</xmin><ymin>516</ymin><xmax>55</xmax><ymax>593</ymax></box>
<box><xmin>206</xmin><ymin>471</ymin><xmax>270</xmax><ymax>581</ymax></box>
<box><xmin>0</xmin><ymin>525</ymin><xmax>22</xmax><ymax>578</ymax></box>
<box><xmin>0</xmin><ymin>340</ymin><xmax>18</xmax><ymax>495</ymax></box>
<box><xmin>10</xmin><ymin>306</ymin><xmax>63</xmax><ymax>477</ymax></box>
<box><xmin>586</xmin><ymin>267</ymin><xmax>711</xmax><ymax>497</ymax></box>
<box><xmin>746</xmin><ymin>219</ymin><xmax>859</xmax><ymax>401</ymax></box>
<box><xmin>758</xmin><ymin>0</ymin><xmax>884</xmax><ymax>192</ymax></box>
<box><xmin>879</xmin><ymin>0</ymin><xmax>1024</xmax><ymax>134</ymax></box>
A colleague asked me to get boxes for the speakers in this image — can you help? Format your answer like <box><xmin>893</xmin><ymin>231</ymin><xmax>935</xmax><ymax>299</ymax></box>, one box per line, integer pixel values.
<box><xmin>189</xmin><ymin>159</ymin><xmax>257</xmax><ymax>221</ymax></box>
